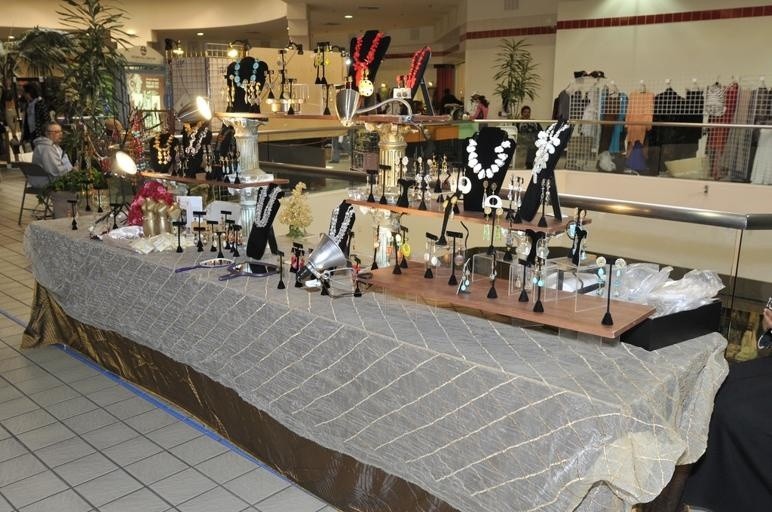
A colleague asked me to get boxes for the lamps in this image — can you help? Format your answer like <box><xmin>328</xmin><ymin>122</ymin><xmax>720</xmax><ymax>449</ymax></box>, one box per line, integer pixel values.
<box><xmin>172</xmin><ymin>40</ymin><xmax>184</xmax><ymax>55</ymax></box>
<box><xmin>174</xmin><ymin>95</ymin><xmax>214</xmax><ymax>123</ymax></box>
<box><xmin>227</xmin><ymin>40</ymin><xmax>251</xmax><ymax>63</ymax></box>
<box><xmin>109</xmin><ymin>150</ymin><xmax>137</xmax><ymax>179</ymax></box>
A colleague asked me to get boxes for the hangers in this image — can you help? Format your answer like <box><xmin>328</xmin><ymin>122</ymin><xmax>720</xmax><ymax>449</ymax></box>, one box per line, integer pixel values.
<box><xmin>553</xmin><ymin>73</ymin><xmax>771</xmax><ymax>103</ymax></box>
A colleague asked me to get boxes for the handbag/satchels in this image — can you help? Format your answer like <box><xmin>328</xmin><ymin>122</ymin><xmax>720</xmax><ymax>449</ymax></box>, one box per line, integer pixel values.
<box><xmin>475</xmin><ymin>111</ymin><xmax>483</xmax><ymax>119</ymax></box>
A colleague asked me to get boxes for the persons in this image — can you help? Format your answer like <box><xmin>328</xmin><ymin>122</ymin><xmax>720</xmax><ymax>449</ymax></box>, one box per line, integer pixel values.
<box><xmin>28</xmin><ymin>122</ymin><xmax>75</xmax><ymax>192</ymax></box>
<box><xmin>22</xmin><ymin>85</ymin><xmax>51</xmax><ymax>151</ymax></box>
<box><xmin>644</xmin><ymin>290</ymin><xmax>770</xmax><ymax>511</ymax></box>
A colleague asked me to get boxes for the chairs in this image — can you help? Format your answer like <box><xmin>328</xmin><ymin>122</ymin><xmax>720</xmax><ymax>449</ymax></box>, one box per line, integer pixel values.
<box><xmin>16</xmin><ymin>162</ymin><xmax>56</xmax><ymax>225</ymax></box>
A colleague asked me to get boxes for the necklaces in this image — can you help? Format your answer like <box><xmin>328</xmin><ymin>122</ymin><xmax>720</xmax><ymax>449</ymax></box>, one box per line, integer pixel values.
<box><xmin>82</xmin><ymin>32</ymin><xmax>589</xmax><ymax>292</ymax></box>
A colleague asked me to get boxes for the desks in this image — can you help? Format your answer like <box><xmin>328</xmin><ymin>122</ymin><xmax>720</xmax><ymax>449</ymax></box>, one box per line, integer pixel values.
<box><xmin>27</xmin><ymin>211</ymin><xmax>728</xmax><ymax>512</ymax></box>
